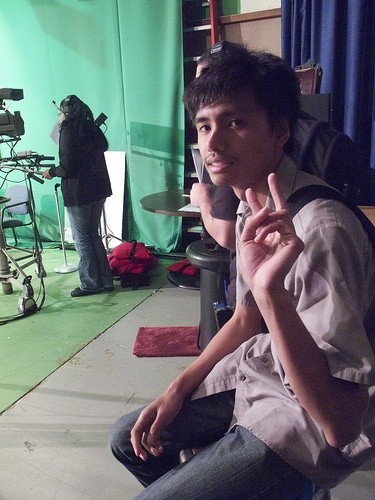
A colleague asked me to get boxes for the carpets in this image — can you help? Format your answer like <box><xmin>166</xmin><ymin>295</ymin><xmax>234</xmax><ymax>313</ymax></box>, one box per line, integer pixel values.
<box><xmin>0</xmin><ymin>248</ymin><xmax>184</xmax><ymax>414</ymax></box>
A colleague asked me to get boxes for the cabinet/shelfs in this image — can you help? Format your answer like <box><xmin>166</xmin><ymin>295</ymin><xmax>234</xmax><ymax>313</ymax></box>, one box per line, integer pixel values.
<box><xmin>183</xmin><ymin>1</ymin><xmax>281</xmax><ymax>202</ymax></box>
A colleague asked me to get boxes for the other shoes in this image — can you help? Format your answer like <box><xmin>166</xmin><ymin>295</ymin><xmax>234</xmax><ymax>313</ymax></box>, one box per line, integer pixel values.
<box><xmin>71</xmin><ymin>287</ymin><xmax>114</xmax><ymax>297</ymax></box>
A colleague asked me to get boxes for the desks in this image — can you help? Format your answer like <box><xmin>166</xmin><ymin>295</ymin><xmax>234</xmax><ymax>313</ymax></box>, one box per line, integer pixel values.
<box><xmin>139</xmin><ymin>190</ymin><xmax>208</xmax><ymax>291</ymax></box>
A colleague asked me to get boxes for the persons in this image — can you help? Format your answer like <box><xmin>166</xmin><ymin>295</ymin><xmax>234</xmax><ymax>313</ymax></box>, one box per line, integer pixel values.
<box><xmin>43</xmin><ymin>95</ymin><xmax>116</xmax><ymax>297</ymax></box>
<box><xmin>110</xmin><ymin>42</ymin><xmax>375</xmax><ymax>500</ymax></box>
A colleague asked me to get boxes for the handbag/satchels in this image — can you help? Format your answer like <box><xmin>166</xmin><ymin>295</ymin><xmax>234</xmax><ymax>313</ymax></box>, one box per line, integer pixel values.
<box><xmin>107</xmin><ymin>239</ymin><xmax>158</xmax><ymax>274</ymax></box>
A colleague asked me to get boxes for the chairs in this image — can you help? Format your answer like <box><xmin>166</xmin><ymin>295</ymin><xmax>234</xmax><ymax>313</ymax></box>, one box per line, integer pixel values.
<box><xmin>0</xmin><ymin>186</ymin><xmax>43</xmax><ymax>263</ymax></box>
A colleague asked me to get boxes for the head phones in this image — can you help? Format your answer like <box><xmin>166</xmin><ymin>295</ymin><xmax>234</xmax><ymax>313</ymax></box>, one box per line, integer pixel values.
<box><xmin>62</xmin><ymin>95</ymin><xmax>73</xmax><ymax>118</ymax></box>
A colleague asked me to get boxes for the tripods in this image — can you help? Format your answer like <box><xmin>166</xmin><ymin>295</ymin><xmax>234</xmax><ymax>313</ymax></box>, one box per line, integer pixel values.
<box><xmin>99</xmin><ymin>206</ymin><xmax>126</xmax><ymax>253</ymax></box>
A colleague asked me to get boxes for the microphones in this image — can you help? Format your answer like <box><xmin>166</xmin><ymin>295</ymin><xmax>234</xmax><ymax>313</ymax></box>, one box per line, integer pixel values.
<box><xmin>52</xmin><ymin>100</ymin><xmax>63</xmax><ymax>113</ymax></box>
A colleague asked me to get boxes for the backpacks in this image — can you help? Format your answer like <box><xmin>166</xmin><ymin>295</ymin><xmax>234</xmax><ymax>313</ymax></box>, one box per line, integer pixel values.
<box><xmin>286</xmin><ymin>106</ymin><xmax>359</xmax><ymax>197</ymax></box>
